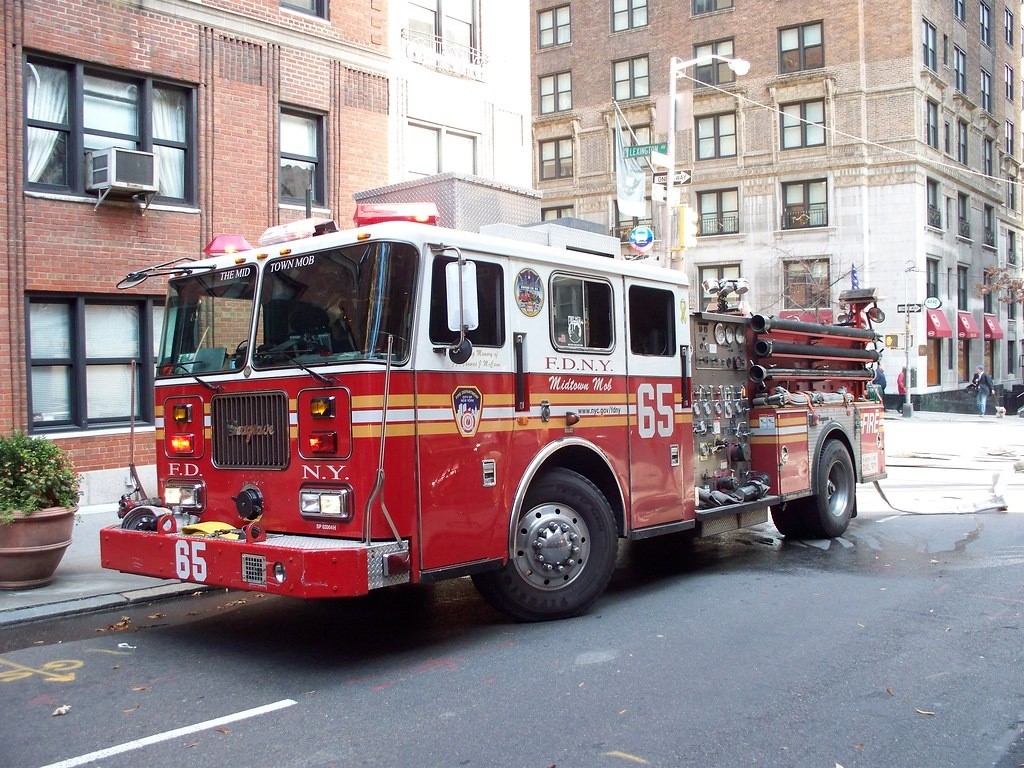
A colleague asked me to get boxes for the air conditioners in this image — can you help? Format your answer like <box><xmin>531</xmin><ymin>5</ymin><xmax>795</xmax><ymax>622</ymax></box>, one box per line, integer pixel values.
<box><xmin>86</xmin><ymin>146</ymin><xmax>160</xmax><ymax>196</ymax></box>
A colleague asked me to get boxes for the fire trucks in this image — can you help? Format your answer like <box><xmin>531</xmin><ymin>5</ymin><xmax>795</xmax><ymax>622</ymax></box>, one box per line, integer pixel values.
<box><xmin>97</xmin><ymin>171</ymin><xmax>888</xmax><ymax>624</ymax></box>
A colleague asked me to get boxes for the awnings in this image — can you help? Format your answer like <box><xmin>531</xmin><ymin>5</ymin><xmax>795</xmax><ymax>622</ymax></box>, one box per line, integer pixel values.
<box><xmin>958</xmin><ymin>314</ymin><xmax>980</xmax><ymax>339</ymax></box>
<box><xmin>984</xmin><ymin>316</ymin><xmax>1004</xmax><ymax>340</ymax></box>
<box><xmin>927</xmin><ymin>309</ymin><xmax>951</xmax><ymax>338</ymax></box>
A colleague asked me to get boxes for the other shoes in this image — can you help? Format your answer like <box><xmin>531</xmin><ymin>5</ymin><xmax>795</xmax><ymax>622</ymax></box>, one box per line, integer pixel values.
<box><xmin>895</xmin><ymin>406</ymin><xmax>903</xmax><ymax>413</ymax></box>
<box><xmin>978</xmin><ymin>413</ymin><xmax>984</xmax><ymax>417</ymax></box>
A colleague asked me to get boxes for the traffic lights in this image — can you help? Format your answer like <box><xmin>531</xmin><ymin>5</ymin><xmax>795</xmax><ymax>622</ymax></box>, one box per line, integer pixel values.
<box><xmin>671</xmin><ymin>202</ymin><xmax>699</xmax><ymax>251</ymax></box>
<box><xmin>885</xmin><ymin>332</ymin><xmax>914</xmax><ymax>351</ymax></box>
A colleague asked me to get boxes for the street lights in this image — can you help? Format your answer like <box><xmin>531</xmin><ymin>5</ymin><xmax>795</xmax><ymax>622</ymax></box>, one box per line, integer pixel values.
<box><xmin>665</xmin><ymin>54</ymin><xmax>751</xmax><ymax>269</ymax></box>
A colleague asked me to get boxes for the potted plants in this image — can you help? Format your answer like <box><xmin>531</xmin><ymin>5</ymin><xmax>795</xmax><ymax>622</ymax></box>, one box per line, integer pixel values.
<box><xmin>0</xmin><ymin>428</ymin><xmax>85</xmax><ymax>592</ymax></box>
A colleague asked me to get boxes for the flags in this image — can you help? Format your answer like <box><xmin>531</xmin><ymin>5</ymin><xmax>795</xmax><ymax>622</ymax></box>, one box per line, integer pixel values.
<box><xmin>615</xmin><ymin>111</ymin><xmax>646</xmax><ymax>218</ymax></box>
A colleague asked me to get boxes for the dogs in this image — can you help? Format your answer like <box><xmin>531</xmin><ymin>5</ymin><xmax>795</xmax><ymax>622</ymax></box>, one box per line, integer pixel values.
<box><xmin>993</xmin><ymin>403</ymin><xmax>1006</xmax><ymax>418</ymax></box>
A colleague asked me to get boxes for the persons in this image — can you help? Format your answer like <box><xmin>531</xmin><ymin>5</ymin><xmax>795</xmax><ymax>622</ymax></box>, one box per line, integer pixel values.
<box><xmin>972</xmin><ymin>365</ymin><xmax>995</xmax><ymax>417</ymax></box>
<box><xmin>896</xmin><ymin>367</ymin><xmax>908</xmax><ymax>413</ymax></box>
<box><xmin>874</xmin><ymin>365</ymin><xmax>887</xmax><ymax>412</ymax></box>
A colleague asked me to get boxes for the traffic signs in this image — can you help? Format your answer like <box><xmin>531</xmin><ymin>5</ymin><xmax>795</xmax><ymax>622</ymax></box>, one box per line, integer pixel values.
<box><xmin>653</xmin><ymin>169</ymin><xmax>692</xmax><ymax>185</ymax></box>
<box><xmin>897</xmin><ymin>304</ymin><xmax>920</xmax><ymax>312</ymax></box>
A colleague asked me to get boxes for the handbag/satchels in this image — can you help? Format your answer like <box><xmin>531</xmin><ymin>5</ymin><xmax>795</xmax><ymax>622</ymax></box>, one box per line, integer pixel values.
<box><xmin>966</xmin><ymin>384</ymin><xmax>979</xmax><ymax>398</ymax></box>
